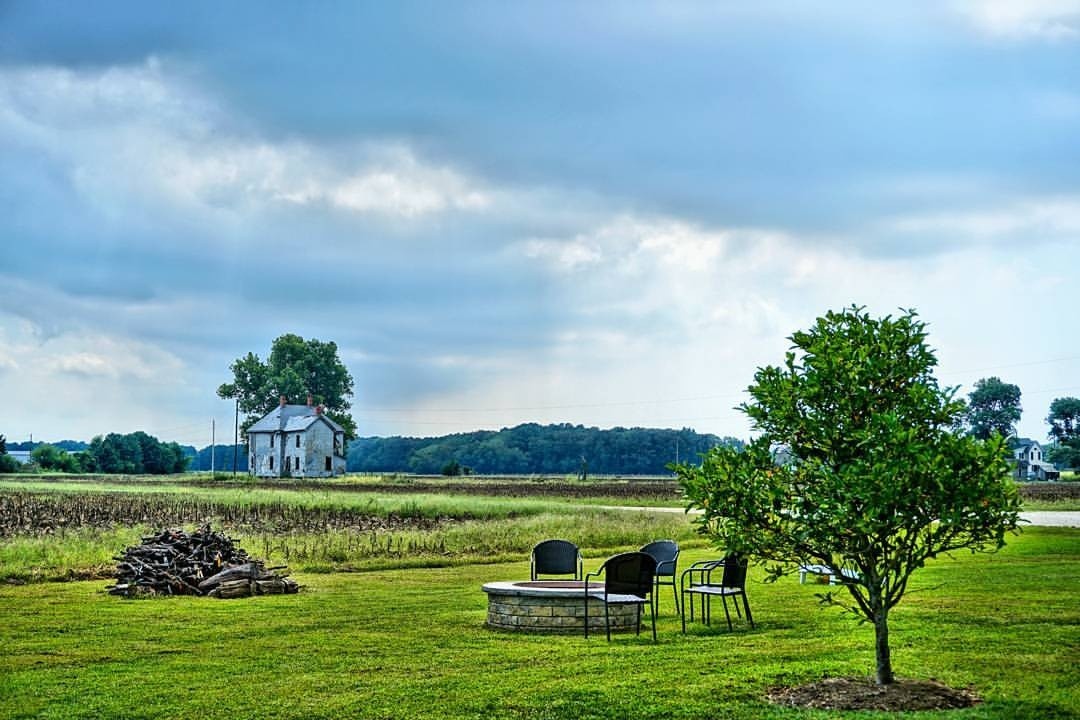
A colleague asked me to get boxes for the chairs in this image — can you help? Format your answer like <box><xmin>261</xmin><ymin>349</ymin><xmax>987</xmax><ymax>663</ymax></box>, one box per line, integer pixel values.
<box><xmin>529</xmin><ymin>539</ymin><xmax>584</xmax><ymax>580</ymax></box>
<box><xmin>681</xmin><ymin>551</ymin><xmax>755</xmax><ymax>633</ymax></box>
<box><xmin>585</xmin><ymin>552</ymin><xmax>659</xmax><ymax>642</ymax></box>
<box><xmin>639</xmin><ymin>539</ymin><xmax>681</xmax><ymax>619</ymax></box>
